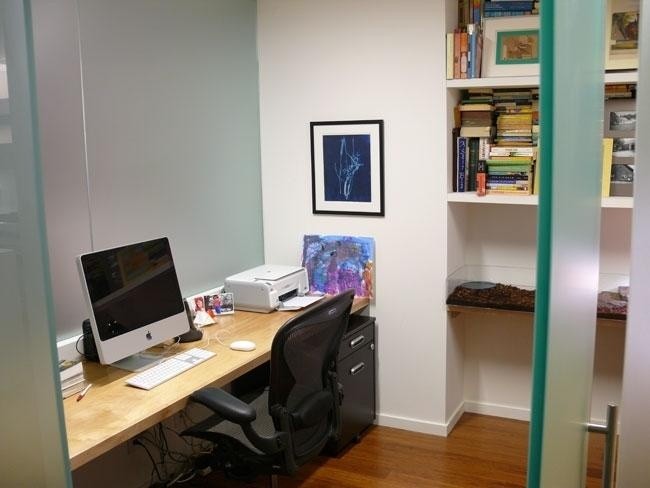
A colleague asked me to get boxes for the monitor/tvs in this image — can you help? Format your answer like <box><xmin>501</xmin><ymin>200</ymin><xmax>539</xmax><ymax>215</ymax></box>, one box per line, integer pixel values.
<box><xmin>75</xmin><ymin>235</ymin><xmax>190</xmax><ymax>373</ymax></box>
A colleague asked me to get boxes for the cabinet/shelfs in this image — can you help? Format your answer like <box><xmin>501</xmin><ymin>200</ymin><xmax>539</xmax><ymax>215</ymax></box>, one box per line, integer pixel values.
<box><xmin>446</xmin><ymin>75</ymin><xmax>632</xmax><ymax>321</ymax></box>
<box><xmin>322</xmin><ymin>316</ymin><xmax>377</xmax><ymax>459</ymax></box>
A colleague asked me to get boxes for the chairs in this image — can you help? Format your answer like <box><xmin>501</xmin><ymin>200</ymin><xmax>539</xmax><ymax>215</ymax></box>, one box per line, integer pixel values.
<box><xmin>178</xmin><ymin>285</ymin><xmax>357</xmax><ymax>486</ymax></box>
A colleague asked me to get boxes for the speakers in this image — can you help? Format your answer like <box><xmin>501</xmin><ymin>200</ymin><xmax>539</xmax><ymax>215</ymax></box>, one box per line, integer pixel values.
<box><xmin>82</xmin><ymin>318</ymin><xmax>100</xmax><ymax>363</ymax></box>
<box><xmin>174</xmin><ymin>298</ymin><xmax>202</xmax><ymax>342</ymax></box>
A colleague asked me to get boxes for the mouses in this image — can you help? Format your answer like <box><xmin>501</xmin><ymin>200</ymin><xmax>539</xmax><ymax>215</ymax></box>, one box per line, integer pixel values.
<box><xmin>230</xmin><ymin>340</ymin><xmax>256</xmax><ymax>351</ymax></box>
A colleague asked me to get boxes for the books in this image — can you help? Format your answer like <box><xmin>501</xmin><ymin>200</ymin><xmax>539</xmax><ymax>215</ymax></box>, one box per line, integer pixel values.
<box><xmin>58</xmin><ymin>359</ymin><xmax>86</xmax><ymax>400</ymax></box>
<box><xmin>445</xmin><ymin>0</ymin><xmax>641</xmax><ymax>198</ymax></box>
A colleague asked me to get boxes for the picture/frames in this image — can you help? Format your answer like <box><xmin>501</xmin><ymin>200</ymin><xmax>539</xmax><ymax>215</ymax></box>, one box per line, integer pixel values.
<box><xmin>482</xmin><ymin>15</ymin><xmax>540</xmax><ymax>78</ymax></box>
<box><xmin>310</xmin><ymin>118</ymin><xmax>386</xmax><ymax>217</ymax></box>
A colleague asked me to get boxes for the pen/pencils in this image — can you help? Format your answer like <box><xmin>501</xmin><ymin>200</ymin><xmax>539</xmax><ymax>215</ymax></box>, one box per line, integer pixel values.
<box><xmin>77</xmin><ymin>383</ymin><xmax>93</xmax><ymax>401</ymax></box>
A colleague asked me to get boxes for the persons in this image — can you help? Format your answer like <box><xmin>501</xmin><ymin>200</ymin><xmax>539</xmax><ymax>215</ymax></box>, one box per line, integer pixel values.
<box><xmin>213</xmin><ymin>294</ymin><xmax>222</xmax><ymax>314</ymax></box>
<box><xmin>195</xmin><ymin>297</ymin><xmax>204</xmax><ymax>310</ymax></box>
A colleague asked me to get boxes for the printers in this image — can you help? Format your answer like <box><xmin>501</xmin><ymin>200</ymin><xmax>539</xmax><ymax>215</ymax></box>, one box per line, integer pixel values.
<box><xmin>224</xmin><ymin>264</ymin><xmax>326</xmax><ymax>314</ymax></box>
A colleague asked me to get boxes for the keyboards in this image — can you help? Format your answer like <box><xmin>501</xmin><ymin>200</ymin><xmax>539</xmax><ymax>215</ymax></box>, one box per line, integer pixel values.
<box><xmin>125</xmin><ymin>347</ymin><xmax>217</xmax><ymax>392</ymax></box>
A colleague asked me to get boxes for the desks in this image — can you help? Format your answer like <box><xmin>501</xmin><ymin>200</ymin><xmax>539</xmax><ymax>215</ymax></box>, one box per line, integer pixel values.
<box><xmin>68</xmin><ymin>286</ymin><xmax>377</xmax><ymax>472</ymax></box>
